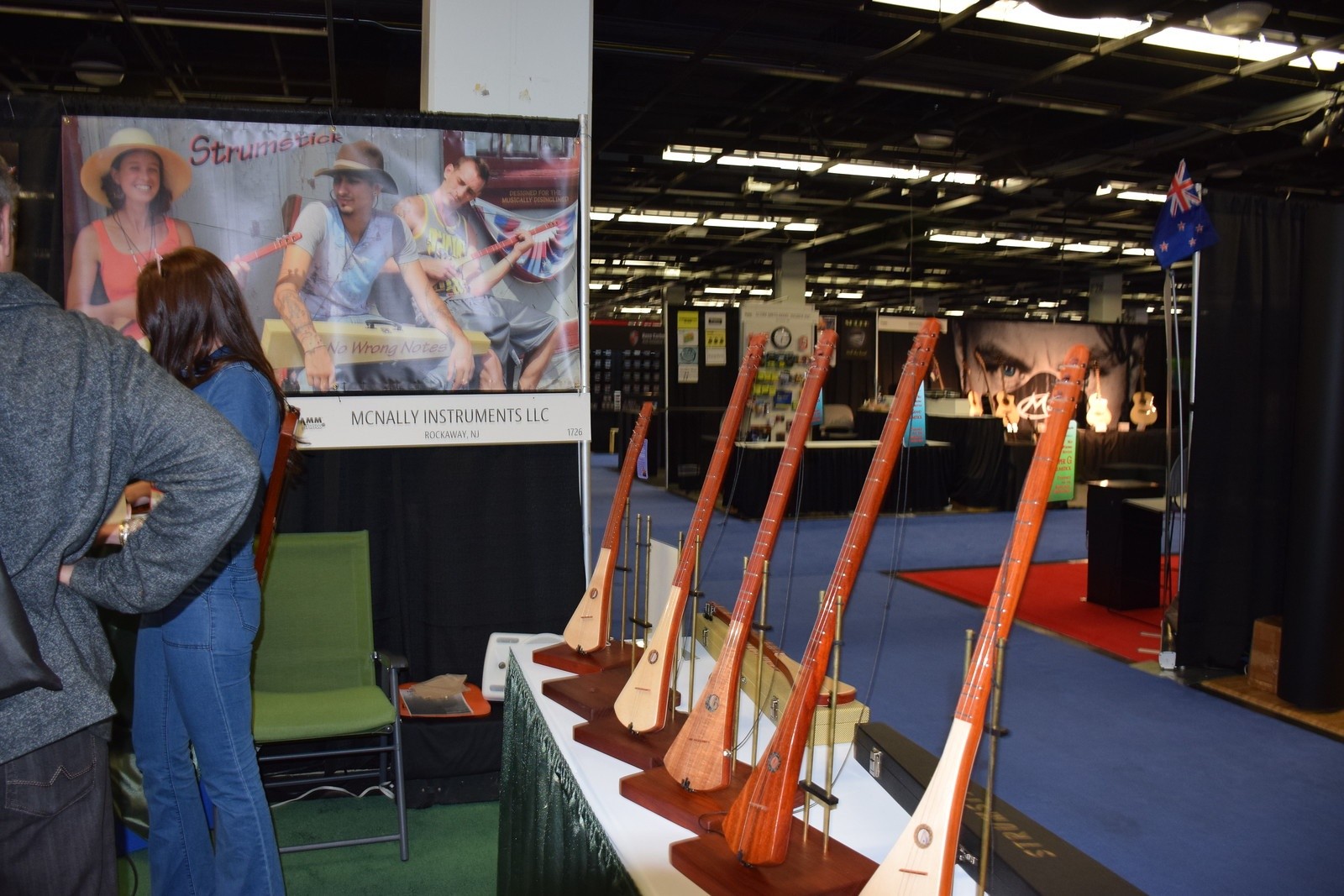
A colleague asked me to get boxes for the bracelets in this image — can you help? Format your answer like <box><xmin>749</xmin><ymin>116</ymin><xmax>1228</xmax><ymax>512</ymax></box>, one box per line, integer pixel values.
<box><xmin>120</xmin><ymin>518</ymin><xmax>145</xmax><ymax>544</ymax></box>
<box><xmin>505</xmin><ymin>257</ymin><xmax>514</xmax><ymax>269</ymax></box>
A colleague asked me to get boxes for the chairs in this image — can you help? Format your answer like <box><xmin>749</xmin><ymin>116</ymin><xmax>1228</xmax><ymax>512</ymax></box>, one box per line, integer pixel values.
<box><xmin>252</xmin><ymin>531</ymin><xmax>409</xmax><ymax>863</ymax></box>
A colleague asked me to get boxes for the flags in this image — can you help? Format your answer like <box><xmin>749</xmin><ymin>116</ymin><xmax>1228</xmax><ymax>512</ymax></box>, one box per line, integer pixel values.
<box><xmin>1152</xmin><ymin>159</ymin><xmax>1218</xmax><ymax>269</ymax></box>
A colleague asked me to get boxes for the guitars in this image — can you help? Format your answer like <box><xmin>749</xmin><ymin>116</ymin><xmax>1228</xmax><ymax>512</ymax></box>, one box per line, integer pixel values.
<box><xmin>974</xmin><ymin>350</ymin><xmax>1008</xmax><ymax>441</ymax></box>
<box><xmin>1086</xmin><ymin>363</ymin><xmax>1112</xmax><ymax>426</ymax></box>
<box><xmin>1130</xmin><ymin>355</ymin><xmax>1158</xmax><ymax>426</ymax></box>
<box><xmin>993</xmin><ymin>359</ymin><xmax>1020</xmax><ymax>428</ymax></box>
<box><xmin>960</xmin><ymin>359</ymin><xmax>984</xmax><ymax>417</ymax></box>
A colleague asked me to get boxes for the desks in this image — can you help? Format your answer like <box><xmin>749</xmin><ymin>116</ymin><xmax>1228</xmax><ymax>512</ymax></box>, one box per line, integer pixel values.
<box><xmin>738</xmin><ymin>439</ymin><xmax>953</xmax><ymax>515</ymax></box>
<box><xmin>1079</xmin><ymin>430</ymin><xmax>1184</xmax><ymax>485</ymax></box>
<box><xmin>499</xmin><ymin>638</ymin><xmax>988</xmax><ymax>896</ymax></box>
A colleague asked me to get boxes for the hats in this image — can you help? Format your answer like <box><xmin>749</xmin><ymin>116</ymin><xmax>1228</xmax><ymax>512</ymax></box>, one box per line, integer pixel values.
<box><xmin>310</xmin><ymin>139</ymin><xmax>401</xmax><ymax>196</ymax></box>
<box><xmin>80</xmin><ymin>125</ymin><xmax>192</xmax><ymax>208</ymax></box>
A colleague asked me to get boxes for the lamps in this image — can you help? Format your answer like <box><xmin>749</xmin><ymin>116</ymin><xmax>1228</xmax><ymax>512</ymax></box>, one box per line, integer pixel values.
<box><xmin>71</xmin><ymin>61</ymin><xmax>123</xmax><ymax>87</ymax></box>
<box><xmin>594</xmin><ymin>0</ymin><xmax>1344</xmax><ymax>323</ymax></box>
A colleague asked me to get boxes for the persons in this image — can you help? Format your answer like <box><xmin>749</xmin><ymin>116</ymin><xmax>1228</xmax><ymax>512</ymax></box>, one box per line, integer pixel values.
<box><xmin>273</xmin><ymin>142</ymin><xmax>476</xmax><ymax>395</ymax></box>
<box><xmin>380</xmin><ymin>155</ymin><xmax>562</xmax><ymax>390</ymax></box>
<box><xmin>0</xmin><ymin>151</ymin><xmax>260</xmax><ymax>896</ymax></box>
<box><xmin>64</xmin><ymin>129</ymin><xmax>251</xmax><ymax>351</ymax></box>
<box><xmin>953</xmin><ymin>320</ymin><xmax>1148</xmax><ymax>431</ymax></box>
<box><xmin>132</xmin><ymin>248</ymin><xmax>287</xmax><ymax>896</ymax></box>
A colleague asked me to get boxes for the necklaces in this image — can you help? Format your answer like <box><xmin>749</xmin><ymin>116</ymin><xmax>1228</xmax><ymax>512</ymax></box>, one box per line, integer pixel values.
<box><xmin>431</xmin><ymin>192</ymin><xmax>458</xmax><ymax>235</ymax></box>
<box><xmin>112</xmin><ymin>209</ymin><xmax>156</xmax><ymax>271</ymax></box>
<box><xmin>304</xmin><ymin>344</ymin><xmax>327</xmax><ymax>354</ymax></box>
